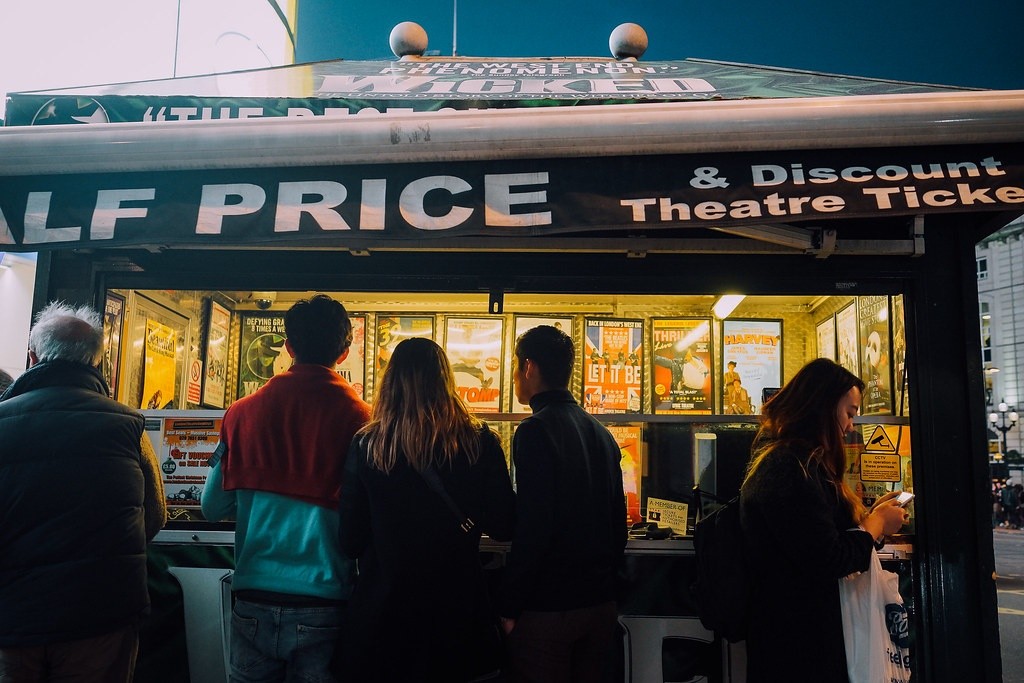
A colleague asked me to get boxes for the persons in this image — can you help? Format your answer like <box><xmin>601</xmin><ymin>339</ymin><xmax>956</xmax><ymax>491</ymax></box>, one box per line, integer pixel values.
<box><xmin>555</xmin><ymin>322</ymin><xmax>562</xmax><ymax>331</ymax></box>
<box><xmin>629</xmin><ymin>348</ymin><xmax>640</xmax><ymax>375</ymax></box>
<box><xmin>903</xmin><ymin>460</ymin><xmax>913</xmax><ymax>519</ymax></box>
<box><xmin>202</xmin><ymin>293</ymin><xmax>377</xmax><ymax>683</ymax></box>
<box><xmin>724</xmin><ymin>361</ymin><xmax>741</xmax><ymax>395</ymax></box>
<box><xmin>726</xmin><ymin>379</ymin><xmax>752</xmax><ymax>415</ymax></box>
<box><xmin>0</xmin><ymin>298</ymin><xmax>167</xmax><ymax>683</ymax></box>
<box><xmin>601</xmin><ymin>348</ymin><xmax>611</xmax><ymax>373</ymax></box>
<box><xmin>493</xmin><ymin>325</ymin><xmax>630</xmax><ymax>683</ymax></box>
<box><xmin>654</xmin><ymin>341</ymin><xmax>709</xmax><ymax>410</ymax></box>
<box><xmin>847</xmin><ymin>462</ymin><xmax>866</xmax><ymax>502</ymax></box>
<box><xmin>336</xmin><ymin>337</ymin><xmax>519</xmax><ymax>683</ymax></box>
<box><xmin>618</xmin><ymin>348</ymin><xmax>624</xmax><ymax>364</ymax></box>
<box><xmin>591</xmin><ymin>348</ymin><xmax>602</xmax><ymax>374</ymax></box>
<box><xmin>738</xmin><ymin>357</ymin><xmax>912</xmax><ymax>683</ymax></box>
<box><xmin>992</xmin><ymin>479</ymin><xmax>1024</xmax><ymax>530</ymax></box>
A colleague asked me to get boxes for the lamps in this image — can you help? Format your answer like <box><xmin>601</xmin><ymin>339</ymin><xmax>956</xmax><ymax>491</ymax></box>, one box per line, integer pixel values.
<box><xmin>710</xmin><ymin>293</ymin><xmax>748</xmax><ymax>322</ymax></box>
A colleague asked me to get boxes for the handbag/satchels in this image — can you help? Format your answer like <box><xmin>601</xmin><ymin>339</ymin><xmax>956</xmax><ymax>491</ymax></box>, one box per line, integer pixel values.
<box><xmin>839</xmin><ymin>525</ymin><xmax>911</xmax><ymax>683</ymax></box>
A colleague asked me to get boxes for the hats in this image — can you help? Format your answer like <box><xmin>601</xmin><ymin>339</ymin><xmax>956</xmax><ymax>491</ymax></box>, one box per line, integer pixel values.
<box><xmin>258</xmin><ymin>335</ymin><xmax>285</xmax><ymax>357</ymax></box>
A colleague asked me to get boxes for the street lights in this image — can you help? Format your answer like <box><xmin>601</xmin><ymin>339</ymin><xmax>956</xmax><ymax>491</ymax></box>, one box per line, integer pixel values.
<box><xmin>988</xmin><ymin>396</ymin><xmax>1021</xmax><ymax>481</ymax></box>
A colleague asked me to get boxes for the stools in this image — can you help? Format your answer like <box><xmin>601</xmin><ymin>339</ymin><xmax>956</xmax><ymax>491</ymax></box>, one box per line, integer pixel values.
<box><xmin>616</xmin><ymin>596</ymin><xmax>715</xmax><ymax>683</ymax></box>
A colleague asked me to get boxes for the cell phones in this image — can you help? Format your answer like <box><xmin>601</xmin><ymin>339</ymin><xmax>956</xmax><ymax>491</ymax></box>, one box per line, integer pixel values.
<box><xmin>894</xmin><ymin>491</ymin><xmax>915</xmax><ymax>508</ymax></box>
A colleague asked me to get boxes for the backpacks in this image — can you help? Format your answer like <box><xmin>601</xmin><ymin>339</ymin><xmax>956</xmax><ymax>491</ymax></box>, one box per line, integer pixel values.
<box><xmin>688</xmin><ymin>485</ymin><xmax>762</xmax><ymax>645</ymax></box>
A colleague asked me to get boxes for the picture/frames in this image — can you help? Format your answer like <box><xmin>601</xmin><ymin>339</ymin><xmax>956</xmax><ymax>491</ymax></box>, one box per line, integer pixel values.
<box><xmin>200</xmin><ymin>295</ymin><xmax>232</xmax><ymax>411</ymax></box>
<box><xmin>235</xmin><ymin>312</ymin><xmax>296</xmax><ymax>401</ymax></box>
<box><xmin>335</xmin><ymin>312</ymin><xmax>368</xmax><ymax>404</ymax></box>
<box><xmin>814</xmin><ymin>295</ymin><xmax>910</xmax><ymax>419</ymax></box>
<box><xmin>719</xmin><ymin>317</ymin><xmax>784</xmax><ymax>415</ymax></box>
<box><xmin>442</xmin><ymin>314</ymin><xmax>506</xmax><ymax>413</ymax></box>
<box><xmin>581</xmin><ymin>316</ymin><xmax>644</xmax><ymax>414</ymax></box>
<box><xmin>373</xmin><ymin>313</ymin><xmax>437</xmax><ymax>405</ymax></box>
<box><xmin>649</xmin><ymin>316</ymin><xmax>716</xmax><ymax>416</ymax></box>
<box><xmin>483</xmin><ymin>422</ymin><xmax>520</xmax><ymax>495</ymax></box>
<box><xmin>508</xmin><ymin>313</ymin><xmax>576</xmax><ymax>414</ymax></box>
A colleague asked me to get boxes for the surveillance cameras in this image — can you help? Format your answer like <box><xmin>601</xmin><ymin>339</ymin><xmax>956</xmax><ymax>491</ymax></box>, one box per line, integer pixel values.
<box><xmin>252</xmin><ymin>291</ymin><xmax>277</xmax><ymax>310</ymax></box>
